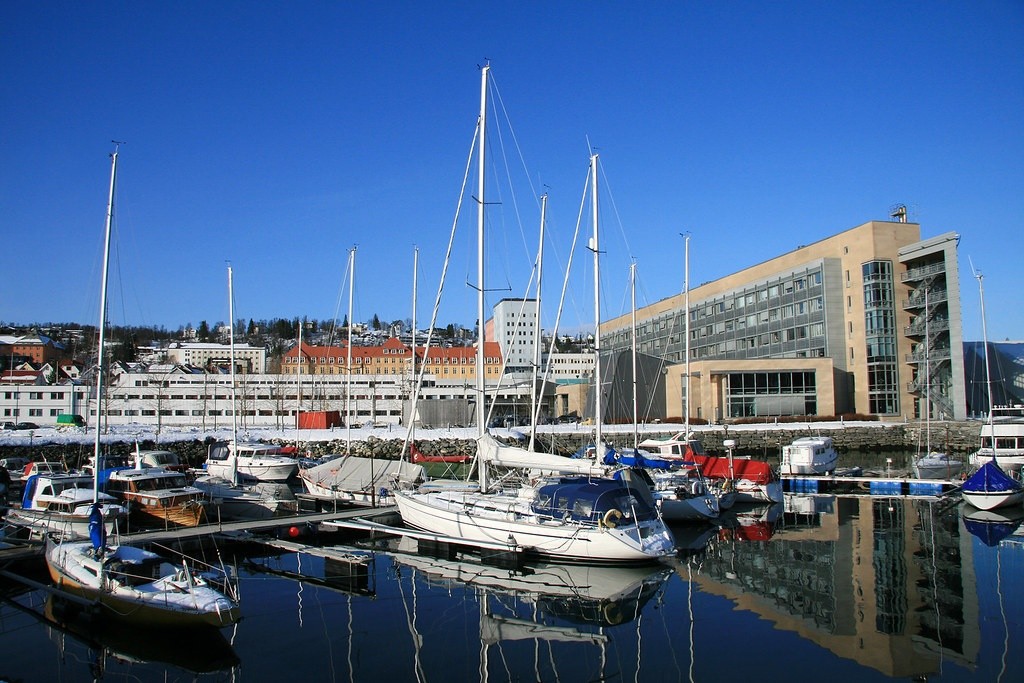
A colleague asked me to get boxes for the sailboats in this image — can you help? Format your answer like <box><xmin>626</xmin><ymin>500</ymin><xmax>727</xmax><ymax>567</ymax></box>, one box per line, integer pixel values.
<box><xmin>569</xmin><ymin>263</ymin><xmax>703</xmax><ymax>480</ymax></box>
<box><xmin>961</xmin><ymin>274</ymin><xmax>1024</xmax><ymax>511</ymax></box>
<box><xmin>297</xmin><ymin>243</ymin><xmax>425</xmax><ymax>507</ymax></box>
<box><xmin>42</xmin><ymin>140</ymin><xmax>246</xmax><ymax>628</ymax></box>
<box><xmin>406</xmin><ymin>241</ymin><xmax>477</xmax><ymax>478</ymax></box>
<box><xmin>474</xmin><ymin>133</ymin><xmax>692</xmax><ymax>484</ymax></box>
<box><xmin>394</xmin><ymin>59</ymin><xmax>680</xmax><ymax>562</ymax></box>
<box><xmin>662</xmin><ymin>237</ymin><xmax>771</xmax><ymax>486</ymax></box>
<box><xmin>191</xmin><ymin>267</ymin><xmax>301</xmax><ymax>486</ymax></box>
<box><xmin>912</xmin><ymin>289</ymin><xmax>963</xmax><ymax>479</ymax></box>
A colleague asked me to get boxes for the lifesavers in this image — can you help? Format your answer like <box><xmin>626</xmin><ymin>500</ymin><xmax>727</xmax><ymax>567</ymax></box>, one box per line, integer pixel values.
<box><xmin>692</xmin><ymin>481</ymin><xmax>702</xmax><ymax>494</ymax></box>
<box><xmin>604</xmin><ymin>508</ymin><xmax>621</xmax><ymax>528</ymax></box>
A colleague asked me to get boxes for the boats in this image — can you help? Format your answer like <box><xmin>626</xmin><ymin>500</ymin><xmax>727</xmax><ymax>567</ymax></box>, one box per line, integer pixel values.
<box><xmin>82</xmin><ymin>429</ymin><xmax>206</xmax><ymax>527</ymax></box>
<box><xmin>296</xmin><ymin>322</ymin><xmax>343</xmax><ymax>471</ymax></box>
<box><xmin>780</xmin><ymin>435</ymin><xmax>838</xmax><ymax>474</ymax></box>
<box><xmin>968</xmin><ymin>399</ymin><xmax>1024</xmax><ymax>474</ymax></box>
<box><xmin>0</xmin><ymin>468</ymin><xmax>116</xmax><ymax>542</ymax></box>
